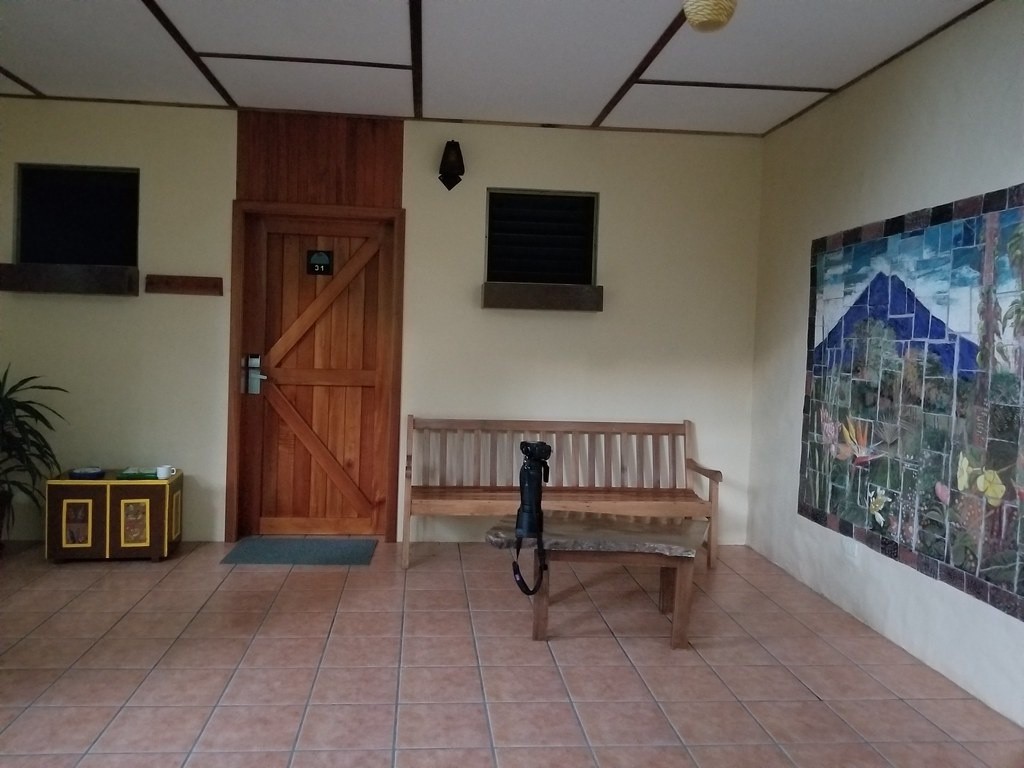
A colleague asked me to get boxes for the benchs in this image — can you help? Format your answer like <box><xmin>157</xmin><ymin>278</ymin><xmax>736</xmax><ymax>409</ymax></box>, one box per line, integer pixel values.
<box><xmin>400</xmin><ymin>414</ymin><xmax>722</xmax><ymax>571</ymax></box>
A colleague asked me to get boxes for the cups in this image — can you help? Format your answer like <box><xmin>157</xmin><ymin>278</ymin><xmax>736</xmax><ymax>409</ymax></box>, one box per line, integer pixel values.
<box><xmin>156</xmin><ymin>465</ymin><xmax>176</xmax><ymax>480</ymax></box>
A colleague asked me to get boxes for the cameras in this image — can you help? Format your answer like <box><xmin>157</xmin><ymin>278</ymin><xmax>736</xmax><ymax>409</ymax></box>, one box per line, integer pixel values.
<box><xmin>515</xmin><ymin>442</ymin><xmax>551</xmax><ymax>538</ymax></box>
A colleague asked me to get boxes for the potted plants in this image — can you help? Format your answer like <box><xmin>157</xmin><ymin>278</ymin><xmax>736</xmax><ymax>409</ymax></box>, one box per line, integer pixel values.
<box><xmin>0</xmin><ymin>361</ymin><xmax>68</xmax><ymax>545</ymax></box>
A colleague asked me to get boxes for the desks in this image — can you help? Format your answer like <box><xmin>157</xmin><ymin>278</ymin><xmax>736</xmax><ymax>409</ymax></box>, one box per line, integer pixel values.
<box><xmin>485</xmin><ymin>510</ymin><xmax>710</xmax><ymax>649</ymax></box>
<box><xmin>45</xmin><ymin>466</ymin><xmax>183</xmax><ymax>563</ymax></box>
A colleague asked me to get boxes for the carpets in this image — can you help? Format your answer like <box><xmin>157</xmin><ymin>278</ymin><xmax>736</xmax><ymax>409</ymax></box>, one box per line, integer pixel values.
<box><xmin>220</xmin><ymin>536</ymin><xmax>380</xmax><ymax>564</ymax></box>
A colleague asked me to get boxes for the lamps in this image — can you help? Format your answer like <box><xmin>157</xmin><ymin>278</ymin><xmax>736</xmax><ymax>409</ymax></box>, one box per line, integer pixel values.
<box><xmin>438</xmin><ymin>140</ymin><xmax>464</xmax><ymax>190</ymax></box>
<box><xmin>682</xmin><ymin>0</ymin><xmax>737</xmax><ymax>32</ymax></box>
